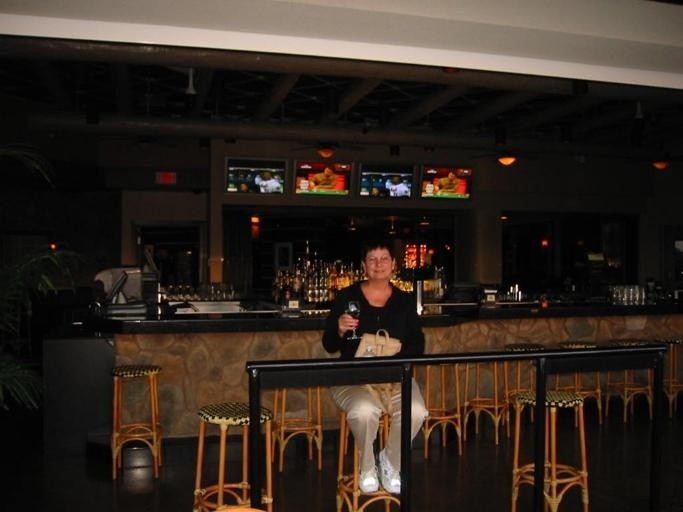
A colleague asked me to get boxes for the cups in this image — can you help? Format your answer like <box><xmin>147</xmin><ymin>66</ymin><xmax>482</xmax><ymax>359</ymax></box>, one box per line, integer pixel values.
<box><xmin>345</xmin><ymin>300</ymin><xmax>364</xmax><ymax>340</ymax></box>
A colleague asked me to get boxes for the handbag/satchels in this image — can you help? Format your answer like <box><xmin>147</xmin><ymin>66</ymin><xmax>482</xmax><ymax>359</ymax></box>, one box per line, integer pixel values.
<box><xmin>356</xmin><ymin>329</ymin><xmax>402</xmax><ymax>413</ymax></box>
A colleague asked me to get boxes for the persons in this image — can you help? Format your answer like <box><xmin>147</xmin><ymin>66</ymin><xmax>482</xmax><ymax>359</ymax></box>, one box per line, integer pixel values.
<box><xmin>390</xmin><ymin>176</ymin><xmax>409</xmax><ymax>196</ymax></box>
<box><xmin>322</xmin><ymin>230</ymin><xmax>426</xmax><ymax>494</ymax></box>
<box><xmin>435</xmin><ymin>171</ymin><xmax>459</xmax><ymax>194</ymax></box>
<box><xmin>260</xmin><ymin>169</ymin><xmax>280</xmax><ymax>193</ymax></box>
<box><xmin>310</xmin><ymin>167</ymin><xmax>338</xmax><ymax>187</ymax></box>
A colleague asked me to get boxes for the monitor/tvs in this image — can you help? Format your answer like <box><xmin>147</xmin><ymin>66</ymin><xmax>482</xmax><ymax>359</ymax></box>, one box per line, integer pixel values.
<box><xmin>357</xmin><ymin>160</ymin><xmax>418</xmax><ymax>201</ymax></box>
<box><xmin>420</xmin><ymin>162</ymin><xmax>475</xmax><ymax>200</ymax></box>
<box><xmin>292</xmin><ymin>158</ymin><xmax>355</xmax><ymax>197</ymax></box>
<box><xmin>223</xmin><ymin>156</ymin><xmax>290</xmax><ymax>196</ymax></box>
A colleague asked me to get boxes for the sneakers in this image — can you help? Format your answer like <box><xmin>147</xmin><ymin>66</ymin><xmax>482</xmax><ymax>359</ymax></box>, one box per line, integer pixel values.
<box><xmin>378</xmin><ymin>448</ymin><xmax>401</xmax><ymax>494</ymax></box>
<box><xmin>358</xmin><ymin>466</ymin><xmax>379</xmax><ymax>493</ymax></box>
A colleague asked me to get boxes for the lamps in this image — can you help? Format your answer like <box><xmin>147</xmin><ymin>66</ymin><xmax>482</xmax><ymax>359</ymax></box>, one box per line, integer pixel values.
<box><xmin>497</xmin><ymin>127</ymin><xmax>519</xmax><ymax>167</ymax></box>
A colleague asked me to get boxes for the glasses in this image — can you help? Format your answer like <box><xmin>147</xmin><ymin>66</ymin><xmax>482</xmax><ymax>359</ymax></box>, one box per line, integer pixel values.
<box><xmin>365</xmin><ymin>255</ymin><xmax>392</xmax><ymax>264</ymax></box>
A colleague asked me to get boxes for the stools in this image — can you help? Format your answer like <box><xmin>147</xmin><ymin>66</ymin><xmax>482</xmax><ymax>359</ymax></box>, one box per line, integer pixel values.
<box><xmin>653</xmin><ymin>336</ymin><xmax>683</xmax><ymax>417</ymax></box>
<box><xmin>336</xmin><ymin>410</ymin><xmax>400</xmax><ymax>512</ymax></box>
<box><xmin>605</xmin><ymin>338</ymin><xmax>653</xmax><ymax>423</ymax></box>
<box><xmin>512</xmin><ymin>391</ymin><xmax>589</xmax><ymax>512</ymax></box>
<box><xmin>193</xmin><ymin>402</ymin><xmax>274</xmax><ymax>512</ymax></box>
<box><xmin>413</xmin><ymin>363</ymin><xmax>462</xmax><ymax>459</ymax></box>
<box><xmin>502</xmin><ymin>343</ymin><xmax>545</xmax><ymax>426</ymax></box>
<box><xmin>555</xmin><ymin>340</ymin><xmax>603</xmax><ymax>425</ymax></box>
<box><xmin>111</xmin><ymin>364</ymin><xmax>163</xmax><ymax>480</ymax></box>
